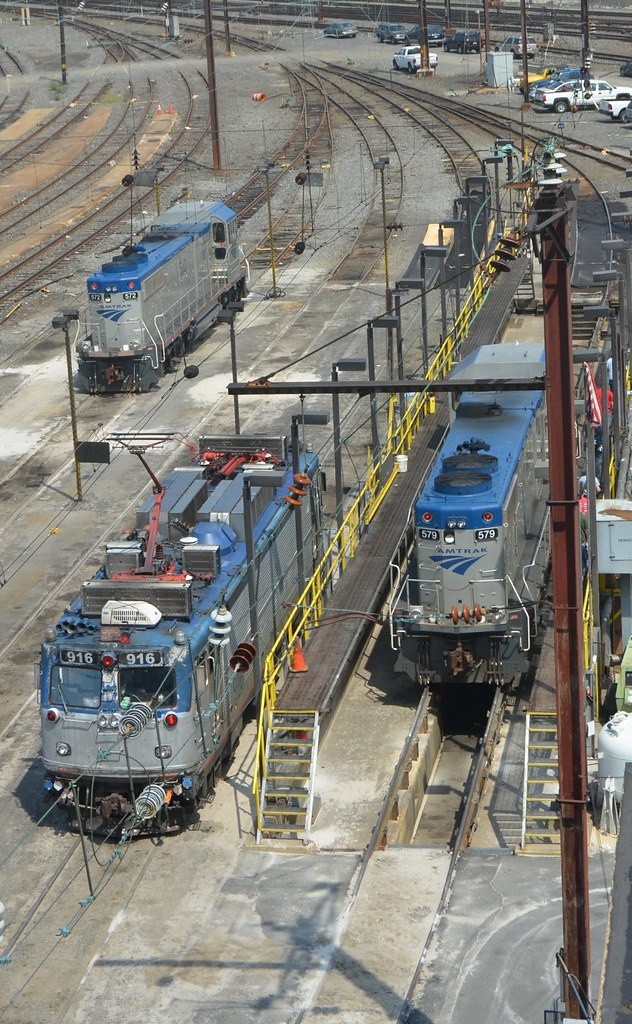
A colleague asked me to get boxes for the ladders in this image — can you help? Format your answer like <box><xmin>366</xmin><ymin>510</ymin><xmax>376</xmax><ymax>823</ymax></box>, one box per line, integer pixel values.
<box><xmin>256</xmin><ymin>710</ymin><xmax>320</xmax><ymax>844</ymax></box>
<box><xmin>521</xmin><ymin>712</ymin><xmax>561</xmax><ymax>850</ymax></box>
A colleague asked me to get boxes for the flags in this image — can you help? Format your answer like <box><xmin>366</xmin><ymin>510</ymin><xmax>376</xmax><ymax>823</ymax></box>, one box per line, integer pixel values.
<box><xmin>582</xmin><ymin>363</ymin><xmax>602</xmax><ymax>429</ymax></box>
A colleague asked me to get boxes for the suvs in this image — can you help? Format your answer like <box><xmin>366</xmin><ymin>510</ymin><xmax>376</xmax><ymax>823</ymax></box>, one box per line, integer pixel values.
<box><xmin>494</xmin><ymin>35</ymin><xmax>539</xmax><ymax>59</ymax></box>
<box><xmin>375</xmin><ymin>23</ymin><xmax>483</xmax><ymax>54</ymax></box>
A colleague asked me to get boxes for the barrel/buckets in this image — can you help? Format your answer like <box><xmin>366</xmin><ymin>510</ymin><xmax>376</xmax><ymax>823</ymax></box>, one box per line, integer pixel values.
<box><xmin>394</xmin><ymin>454</ymin><xmax>409</xmax><ymax>473</ymax></box>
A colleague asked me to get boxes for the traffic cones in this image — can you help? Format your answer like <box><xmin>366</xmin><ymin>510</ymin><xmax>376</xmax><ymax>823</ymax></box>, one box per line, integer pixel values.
<box><xmin>167</xmin><ymin>100</ymin><xmax>174</xmax><ymax>114</ymax></box>
<box><xmin>289</xmin><ymin>636</ymin><xmax>308</xmax><ymax>672</ymax></box>
<box><xmin>157</xmin><ymin>105</ymin><xmax>163</xmax><ymax>115</ymax></box>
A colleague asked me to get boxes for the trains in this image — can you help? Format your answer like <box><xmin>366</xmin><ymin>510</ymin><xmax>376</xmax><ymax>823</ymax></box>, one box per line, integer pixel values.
<box><xmin>390</xmin><ymin>344</ymin><xmax>549</xmax><ymax>686</ymax></box>
<box><xmin>73</xmin><ymin>202</ymin><xmax>250</xmax><ymax>393</ymax></box>
<box><xmin>34</xmin><ymin>434</ymin><xmax>327</xmax><ymax>837</ymax></box>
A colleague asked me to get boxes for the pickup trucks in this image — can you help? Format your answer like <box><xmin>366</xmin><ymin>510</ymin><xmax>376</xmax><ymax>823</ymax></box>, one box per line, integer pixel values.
<box><xmin>392</xmin><ymin>46</ymin><xmax>438</xmax><ymax>73</ymax></box>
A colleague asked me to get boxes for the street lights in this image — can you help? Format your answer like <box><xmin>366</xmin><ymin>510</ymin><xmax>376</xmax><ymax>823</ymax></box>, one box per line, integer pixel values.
<box><xmin>242</xmin><ymin>471</ymin><xmax>286</xmax><ymax>735</ymax></box>
<box><xmin>439</xmin><ymin>220</ymin><xmax>466</xmax><ymax>364</ymax></box>
<box><xmin>466</xmin><ymin>138</ymin><xmax>515</xmax><ymax>310</ymax></box>
<box><xmin>395</xmin><ymin>278</ymin><xmax>425</xmax><ymax>446</ymax></box>
<box><xmin>257</xmin><ymin>160</ymin><xmax>276</xmax><ymax>293</ymax></box>
<box><xmin>367</xmin><ymin>316</ymin><xmax>399</xmax><ymax>497</ymax></box>
<box><xmin>452</xmin><ymin>195</ymin><xmax>477</xmax><ymax>341</ymax></box>
<box><xmin>420</xmin><ymin>247</ymin><xmax>448</xmax><ymax>382</ymax></box>
<box><xmin>52</xmin><ymin>309</ymin><xmax>83</xmax><ymax>500</ymax></box>
<box><xmin>373</xmin><ymin>158</ymin><xmax>389</xmax><ymax>289</ymax></box>
<box><xmin>291</xmin><ymin>408</ymin><xmax>330</xmax><ymax>642</ymax></box>
<box><xmin>217</xmin><ymin>301</ymin><xmax>245</xmax><ymax>433</ymax></box>
<box><xmin>331</xmin><ymin>358</ymin><xmax>367</xmax><ymax>578</ymax></box>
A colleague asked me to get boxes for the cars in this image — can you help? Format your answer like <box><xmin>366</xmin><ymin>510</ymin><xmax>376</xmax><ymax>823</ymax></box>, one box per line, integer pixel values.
<box><xmin>519</xmin><ymin>63</ymin><xmax>632</xmax><ymax>123</ymax></box>
<box><xmin>323</xmin><ymin>22</ymin><xmax>358</xmax><ymax>39</ymax></box>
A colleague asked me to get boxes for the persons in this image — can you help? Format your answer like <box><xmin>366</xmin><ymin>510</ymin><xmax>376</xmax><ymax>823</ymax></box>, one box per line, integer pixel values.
<box><xmin>577</xmin><ymin>357</ymin><xmax>616</xmax><ymax>583</ymax></box>
